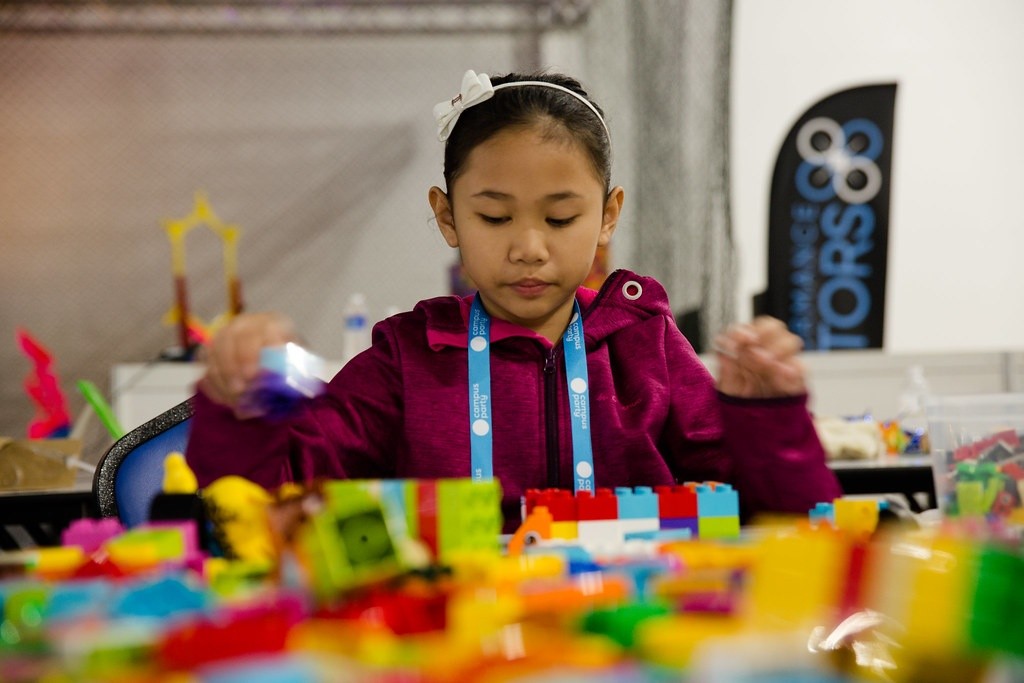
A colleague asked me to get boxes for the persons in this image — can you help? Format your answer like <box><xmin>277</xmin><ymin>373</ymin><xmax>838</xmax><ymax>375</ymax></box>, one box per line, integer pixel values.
<box><xmin>185</xmin><ymin>72</ymin><xmax>836</xmax><ymax>517</ymax></box>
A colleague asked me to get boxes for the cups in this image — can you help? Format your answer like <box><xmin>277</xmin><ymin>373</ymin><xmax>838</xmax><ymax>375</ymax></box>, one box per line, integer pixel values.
<box><xmin>923</xmin><ymin>393</ymin><xmax>1024</xmax><ymax>544</ymax></box>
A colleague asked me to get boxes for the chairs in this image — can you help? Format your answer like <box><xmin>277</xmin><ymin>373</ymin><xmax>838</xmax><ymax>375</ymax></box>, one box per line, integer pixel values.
<box><xmin>90</xmin><ymin>395</ymin><xmax>216</xmax><ymax>537</ymax></box>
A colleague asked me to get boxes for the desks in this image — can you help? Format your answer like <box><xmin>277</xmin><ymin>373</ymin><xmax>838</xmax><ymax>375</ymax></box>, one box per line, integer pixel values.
<box><xmin>0</xmin><ymin>435</ymin><xmax>941</xmax><ymax>552</ymax></box>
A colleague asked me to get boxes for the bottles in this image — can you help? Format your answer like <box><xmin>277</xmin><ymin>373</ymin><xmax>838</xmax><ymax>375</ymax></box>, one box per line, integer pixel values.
<box><xmin>340</xmin><ymin>292</ymin><xmax>369</xmax><ymax>367</ymax></box>
